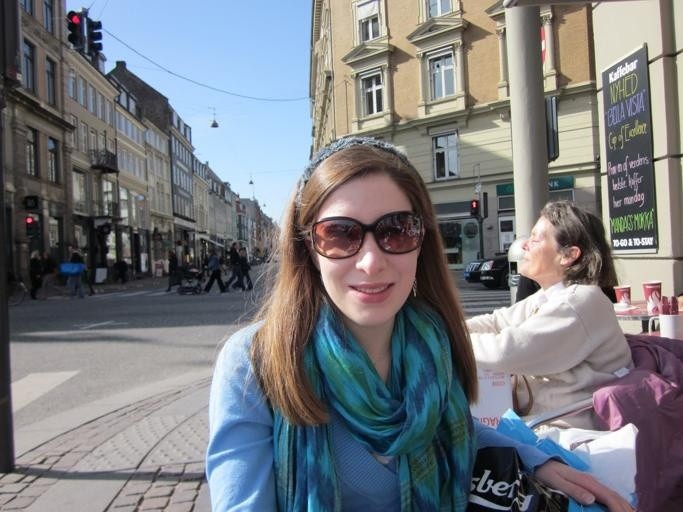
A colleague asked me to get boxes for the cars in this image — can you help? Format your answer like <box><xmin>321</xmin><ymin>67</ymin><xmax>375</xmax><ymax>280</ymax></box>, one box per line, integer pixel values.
<box><xmin>464</xmin><ymin>251</ymin><xmax>509</xmax><ymax>290</ymax></box>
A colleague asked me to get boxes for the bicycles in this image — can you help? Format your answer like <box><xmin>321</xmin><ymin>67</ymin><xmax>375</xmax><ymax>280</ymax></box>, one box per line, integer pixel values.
<box><xmin>9</xmin><ymin>271</ymin><xmax>28</xmax><ymax>308</ymax></box>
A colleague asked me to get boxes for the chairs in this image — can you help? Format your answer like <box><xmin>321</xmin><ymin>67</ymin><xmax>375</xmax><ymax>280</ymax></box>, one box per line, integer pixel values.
<box><xmin>525</xmin><ymin>332</ymin><xmax>657</xmax><ymax>444</ymax></box>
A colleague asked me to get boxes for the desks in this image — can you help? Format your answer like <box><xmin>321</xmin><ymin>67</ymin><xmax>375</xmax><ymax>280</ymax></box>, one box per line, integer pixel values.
<box><xmin>613</xmin><ymin>300</ymin><xmax>683</xmax><ymax>332</ymax></box>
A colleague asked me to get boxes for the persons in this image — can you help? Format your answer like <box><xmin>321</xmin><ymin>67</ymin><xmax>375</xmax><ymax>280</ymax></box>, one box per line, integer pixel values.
<box><xmin>458</xmin><ymin>195</ymin><xmax>636</xmax><ymax>437</ymax></box>
<box><xmin>200</xmin><ymin>134</ymin><xmax>636</xmax><ymax>511</ymax></box>
<box><xmin>75</xmin><ymin>248</ymin><xmax>97</xmax><ymax>297</ymax></box>
<box><xmin>65</xmin><ymin>245</ymin><xmax>85</xmax><ymax>298</ymax></box>
<box><xmin>162</xmin><ymin>250</ymin><xmax>182</xmax><ymax>293</ymax></box>
<box><xmin>231</xmin><ymin>247</ymin><xmax>253</xmax><ymax>290</ymax></box>
<box><xmin>40</xmin><ymin>252</ymin><xmax>59</xmax><ymax>300</ymax></box>
<box><xmin>202</xmin><ymin>246</ymin><xmax>227</xmax><ymax>295</ymax></box>
<box><xmin>224</xmin><ymin>242</ymin><xmax>244</xmax><ymax>290</ymax></box>
<box><xmin>28</xmin><ymin>249</ymin><xmax>43</xmax><ymax>300</ymax></box>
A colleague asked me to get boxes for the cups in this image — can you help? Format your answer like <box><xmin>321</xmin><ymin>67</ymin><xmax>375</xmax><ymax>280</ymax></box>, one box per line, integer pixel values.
<box><xmin>613</xmin><ymin>286</ymin><xmax>631</xmax><ymax>305</ymax></box>
<box><xmin>649</xmin><ymin>314</ymin><xmax>682</xmax><ymax>339</ymax></box>
<box><xmin>642</xmin><ymin>281</ymin><xmax>662</xmax><ymax>305</ymax></box>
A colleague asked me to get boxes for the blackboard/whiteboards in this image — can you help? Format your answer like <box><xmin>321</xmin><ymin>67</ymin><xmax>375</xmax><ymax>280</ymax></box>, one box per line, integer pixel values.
<box><xmin>601</xmin><ymin>43</ymin><xmax>659</xmax><ymax>254</ymax></box>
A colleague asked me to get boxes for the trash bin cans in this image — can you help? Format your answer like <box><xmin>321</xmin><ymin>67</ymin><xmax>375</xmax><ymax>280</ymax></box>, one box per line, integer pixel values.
<box><xmin>94</xmin><ymin>262</ymin><xmax>109</xmax><ymax>284</ymax></box>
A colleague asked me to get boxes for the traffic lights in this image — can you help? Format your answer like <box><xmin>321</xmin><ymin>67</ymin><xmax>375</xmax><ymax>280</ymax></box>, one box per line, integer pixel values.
<box><xmin>66</xmin><ymin>9</ymin><xmax>103</xmax><ymax>57</ymax></box>
<box><xmin>470</xmin><ymin>200</ymin><xmax>479</xmax><ymax>217</ymax></box>
<box><xmin>25</xmin><ymin>213</ymin><xmax>39</xmax><ymax>239</ymax></box>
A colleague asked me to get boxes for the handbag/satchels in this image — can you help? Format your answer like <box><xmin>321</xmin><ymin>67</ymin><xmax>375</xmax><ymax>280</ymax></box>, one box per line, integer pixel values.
<box><xmin>463</xmin><ymin>443</ymin><xmax>573</xmax><ymax>511</ymax></box>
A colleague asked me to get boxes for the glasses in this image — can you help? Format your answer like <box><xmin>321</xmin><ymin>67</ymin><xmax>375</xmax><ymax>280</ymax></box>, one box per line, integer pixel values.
<box><xmin>308</xmin><ymin>211</ymin><xmax>425</xmax><ymax>261</ymax></box>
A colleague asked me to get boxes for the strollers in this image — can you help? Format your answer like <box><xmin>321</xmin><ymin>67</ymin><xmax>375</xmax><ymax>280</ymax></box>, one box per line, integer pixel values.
<box><xmin>177</xmin><ymin>267</ymin><xmax>205</xmax><ymax>295</ymax></box>
